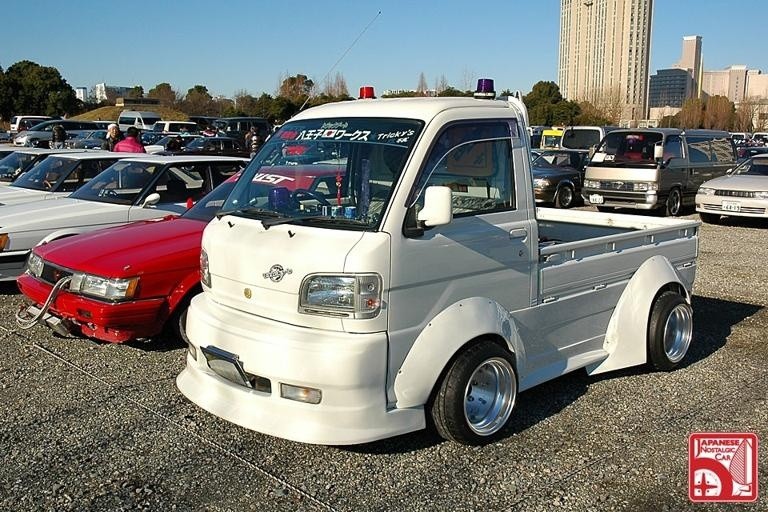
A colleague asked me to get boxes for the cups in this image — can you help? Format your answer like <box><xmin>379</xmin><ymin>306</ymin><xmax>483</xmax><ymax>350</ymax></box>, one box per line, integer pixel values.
<box><xmin>322</xmin><ymin>204</ymin><xmax>358</xmax><ymax>220</ymax></box>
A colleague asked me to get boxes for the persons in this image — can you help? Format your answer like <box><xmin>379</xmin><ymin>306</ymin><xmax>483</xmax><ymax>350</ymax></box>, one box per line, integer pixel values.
<box><xmin>166</xmin><ymin>135</ymin><xmax>183</xmax><ymax>150</ymax></box>
<box><xmin>100</xmin><ymin>124</ymin><xmax>126</xmax><ymax>151</ymax></box>
<box><xmin>203</xmin><ymin>124</ymin><xmax>215</xmax><ymax>137</ymax></box>
<box><xmin>52</xmin><ymin>121</ymin><xmax>67</xmax><ymax>149</ymax></box>
<box><xmin>113</xmin><ymin>127</ymin><xmax>146</xmax><ymax>154</ymax></box>
<box><xmin>245</xmin><ymin>126</ymin><xmax>259</xmax><ymax>149</ymax></box>
<box><xmin>215</xmin><ymin>123</ymin><xmax>230</xmax><ymax>137</ymax></box>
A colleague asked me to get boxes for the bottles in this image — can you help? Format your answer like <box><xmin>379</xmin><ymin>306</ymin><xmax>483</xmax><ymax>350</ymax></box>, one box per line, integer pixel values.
<box><xmin>269</xmin><ymin>187</ymin><xmax>290</xmax><ymax>210</ymax></box>
